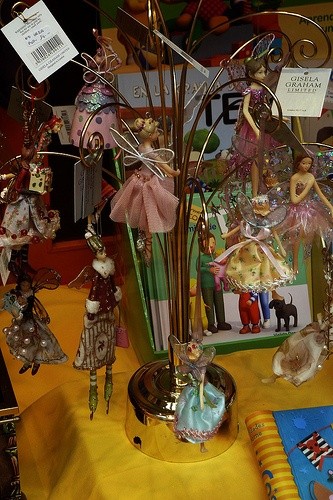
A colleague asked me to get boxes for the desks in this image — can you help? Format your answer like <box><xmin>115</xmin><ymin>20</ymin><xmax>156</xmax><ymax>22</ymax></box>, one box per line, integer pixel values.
<box><xmin>1</xmin><ymin>233</ymin><xmax>333</xmax><ymax>498</ymax></box>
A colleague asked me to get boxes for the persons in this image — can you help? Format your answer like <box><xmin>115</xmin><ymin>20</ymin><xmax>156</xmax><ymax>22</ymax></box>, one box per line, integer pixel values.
<box><xmin>184</xmin><ymin>342</ymin><xmax>209</xmax><ymax>453</ymax></box>
<box><xmin>134</xmin><ymin>117</ymin><xmax>180</xmax><ymax>228</ymax></box>
<box><xmin>240</xmin><ymin>57</ymin><xmax>291</xmax><ymax>206</ymax></box>
<box><xmin>8</xmin><ymin>275</ymin><xmax>41</xmax><ymax>376</ymax></box>
<box><xmin>16</xmin><ymin>144</ymin><xmax>52</xmax><ymax>234</ymax></box>
<box><xmin>261</xmin><ymin>313</ymin><xmax>330</xmax><ymax>388</ymax></box>
<box><xmin>290</xmin><ymin>152</ymin><xmax>333</xmax><ymax>274</ymax></box>
<box><xmin>219</xmin><ymin>198</ymin><xmax>286</xmax><ymax>291</ymax></box>
<box><xmin>69</xmin><ymin>43</ymin><xmax>124</xmax><ymax>149</ymax></box>
<box><xmin>85</xmin><ymin>248</ymin><xmax>122</xmax><ymax>412</ymax></box>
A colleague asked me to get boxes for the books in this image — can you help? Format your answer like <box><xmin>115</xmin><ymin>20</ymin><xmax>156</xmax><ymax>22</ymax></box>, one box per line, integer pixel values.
<box><xmin>242</xmin><ymin>404</ymin><xmax>333</xmax><ymax>500</ymax></box>
